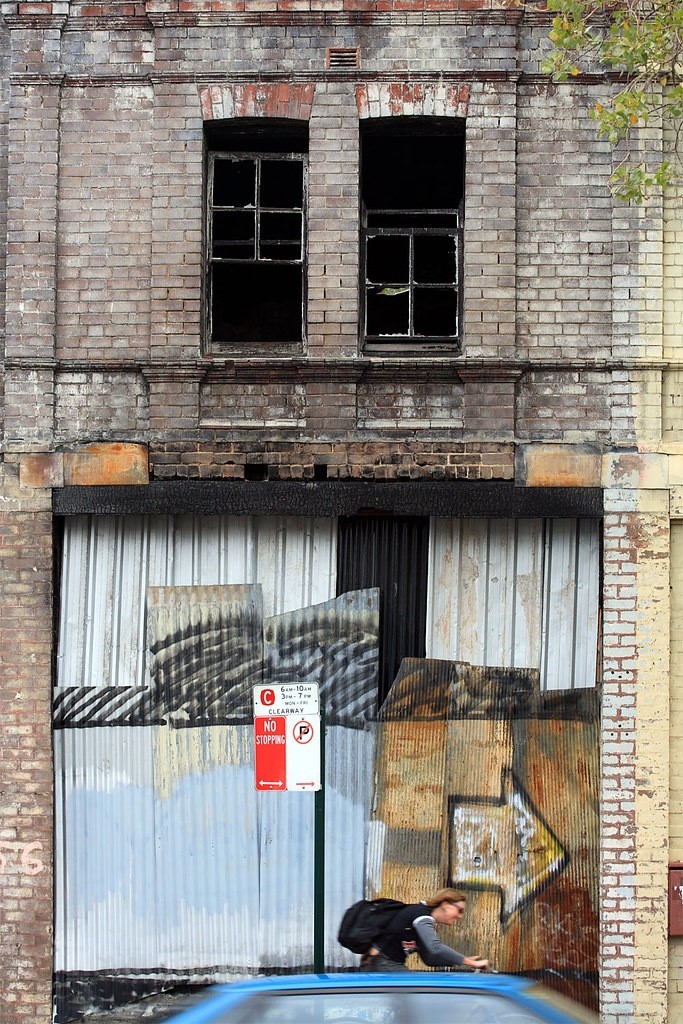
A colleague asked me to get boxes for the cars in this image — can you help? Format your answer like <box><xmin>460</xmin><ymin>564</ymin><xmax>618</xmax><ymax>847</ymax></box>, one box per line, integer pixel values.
<box><xmin>156</xmin><ymin>970</ymin><xmax>605</xmax><ymax>1024</ymax></box>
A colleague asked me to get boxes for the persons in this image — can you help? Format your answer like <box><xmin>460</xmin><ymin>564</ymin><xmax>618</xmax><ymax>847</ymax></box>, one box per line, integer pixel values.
<box><xmin>360</xmin><ymin>888</ymin><xmax>489</xmax><ymax>973</ymax></box>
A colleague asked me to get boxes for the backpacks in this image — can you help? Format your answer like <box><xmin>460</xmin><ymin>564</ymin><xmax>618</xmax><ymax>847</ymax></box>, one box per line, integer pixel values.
<box><xmin>338</xmin><ymin>898</ymin><xmax>422</xmax><ymax>954</ymax></box>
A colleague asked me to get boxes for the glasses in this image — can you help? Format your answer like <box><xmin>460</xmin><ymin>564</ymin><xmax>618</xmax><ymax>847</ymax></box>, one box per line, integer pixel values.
<box><xmin>450</xmin><ymin>902</ymin><xmax>464</xmax><ymax>913</ymax></box>
